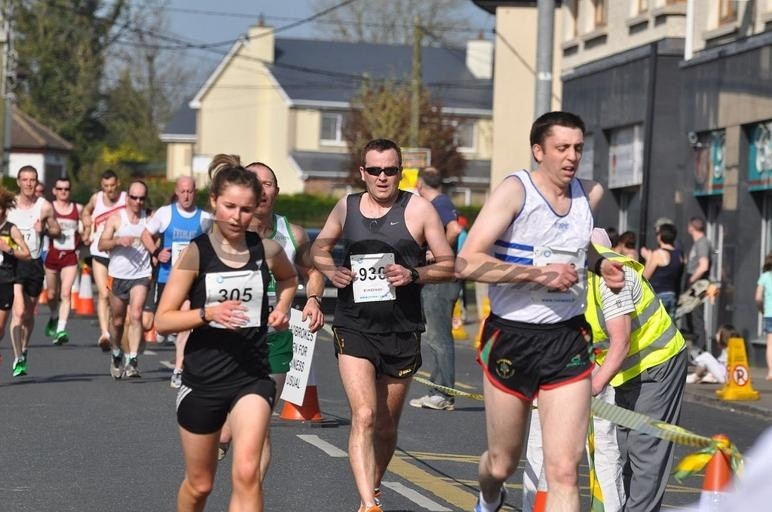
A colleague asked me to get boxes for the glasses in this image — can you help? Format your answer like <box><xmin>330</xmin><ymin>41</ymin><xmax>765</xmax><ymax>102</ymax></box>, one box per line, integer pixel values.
<box><xmin>129</xmin><ymin>194</ymin><xmax>145</xmax><ymax>200</ymax></box>
<box><xmin>55</xmin><ymin>187</ymin><xmax>72</xmax><ymax>191</ymax></box>
<box><xmin>362</xmin><ymin>167</ymin><xmax>403</xmax><ymax>177</ymax></box>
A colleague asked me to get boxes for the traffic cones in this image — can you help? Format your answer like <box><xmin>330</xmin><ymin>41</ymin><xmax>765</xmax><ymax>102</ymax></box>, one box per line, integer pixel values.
<box><xmin>718</xmin><ymin>336</ymin><xmax>760</xmax><ymax>400</ymax></box>
<box><xmin>70</xmin><ymin>274</ymin><xmax>79</xmax><ymax>309</ymax></box>
<box><xmin>76</xmin><ymin>268</ymin><xmax>95</xmax><ymax>315</ymax></box>
<box><xmin>696</xmin><ymin>435</ymin><xmax>742</xmax><ymax>511</ymax></box>
<box><xmin>474</xmin><ymin>297</ymin><xmax>491</xmax><ymax>348</ymax></box>
<box><xmin>452</xmin><ymin>299</ymin><xmax>469</xmax><ymax>340</ymax></box>
<box><xmin>532</xmin><ymin>463</ymin><xmax>548</xmax><ymax>512</ymax></box>
<box><xmin>145</xmin><ymin>323</ymin><xmax>157</xmax><ymax>342</ymax></box>
<box><xmin>37</xmin><ymin>277</ymin><xmax>48</xmax><ymax>305</ymax></box>
<box><xmin>279</xmin><ymin>366</ymin><xmax>326</xmax><ymax>423</ymax></box>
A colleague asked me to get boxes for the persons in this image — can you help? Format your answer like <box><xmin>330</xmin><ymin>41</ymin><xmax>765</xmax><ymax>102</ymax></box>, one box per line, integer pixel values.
<box><xmin>752</xmin><ymin>255</ymin><xmax>771</xmax><ymax>381</ymax></box>
<box><xmin>308</xmin><ymin>139</ymin><xmax>454</xmax><ymax>511</ymax></box>
<box><xmin>583</xmin><ymin>243</ymin><xmax>688</xmax><ymax>511</ymax></box>
<box><xmin>153</xmin><ymin>153</ymin><xmax>300</xmax><ymax>511</ymax></box>
<box><xmin>607</xmin><ymin>216</ymin><xmax>739</xmax><ymax>383</ymax></box>
<box><xmin>408</xmin><ymin>166</ymin><xmax>463</xmax><ymax>411</ymax></box>
<box><xmin>455</xmin><ymin>213</ymin><xmax>469</xmax><ymax>333</ymax></box>
<box><xmin>0</xmin><ymin>155</ymin><xmax>239</xmax><ymax>388</ymax></box>
<box><xmin>453</xmin><ymin>112</ymin><xmax>626</xmax><ymax>512</ymax></box>
<box><xmin>218</xmin><ymin>161</ymin><xmax>326</xmax><ymax>484</ymax></box>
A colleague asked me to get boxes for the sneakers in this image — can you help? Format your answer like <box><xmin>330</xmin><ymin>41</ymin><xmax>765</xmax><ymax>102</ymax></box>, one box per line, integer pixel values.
<box><xmin>409</xmin><ymin>395</ymin><xmax>429</xmax><ymax>408</ymax></box>
<box><xmin>357</xmin><ymin>489</ymin><xmax>384</xmax><ymax>512</ymax></box>
<box><xmin>474</xmin><ymin>482</ymin><xmax>509</xmax><ymax>512</ymax></box>
<box><xmin>699</xmin><ymin>372</ymin><xmax>717</xmax><ymax>382</ymax></box>
<box><xmin>12</xmin><ymin>316</ymin><xmax>184</xmax><ymax>388</ymax></box>
<box><xmin>423</xmin><ymin>394</ymin><xmax>456</xmax><ymax>411</ymax></box>
<box><xmin>218</xmin><ymin>442</ymin><xmax>229</xmax><ymax>462</ymax></box>
<box><xmin>685</xmin><ymin>374</ymin><xmax>699</xmax><ymax>384</ymax></box>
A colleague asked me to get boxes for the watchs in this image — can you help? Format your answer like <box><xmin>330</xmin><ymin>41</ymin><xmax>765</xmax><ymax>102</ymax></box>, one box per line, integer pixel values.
<box><xmin>309</xmin><ymin>295</ymin><xmax>323</xmax><ymax>305</ymax></box>
<box><xmin>407</xmin><ymin>266</ymin><xmax>419</xmax><ymax>284</ymax></box>
<box><xmin>199</xmin><ymin>303</ymin><xmax>210</xmax><ymax>326</ymax></box>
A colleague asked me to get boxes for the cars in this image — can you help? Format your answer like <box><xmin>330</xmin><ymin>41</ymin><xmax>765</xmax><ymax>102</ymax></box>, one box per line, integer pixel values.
<box><xmin>282</xmin><ymin>230</ymin><xmax>343</xmax><ymax>299</ymax></box>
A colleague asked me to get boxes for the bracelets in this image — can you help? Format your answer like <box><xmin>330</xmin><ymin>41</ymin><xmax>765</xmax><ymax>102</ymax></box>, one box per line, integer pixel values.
<box><xmin>594</xmin><ymin>258</ymin><xmax>606</xmax><ymax>277</ymax></box>
